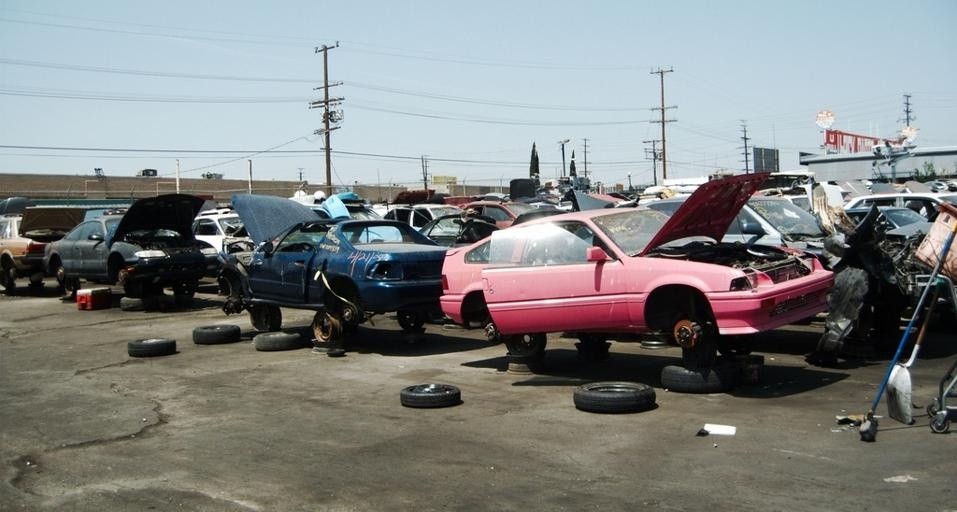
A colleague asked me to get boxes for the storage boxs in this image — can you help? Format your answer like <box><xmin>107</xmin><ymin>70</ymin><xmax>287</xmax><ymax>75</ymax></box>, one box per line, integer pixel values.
<box><xmin>76</xmin><ymin>286</ymin><xmax>113</xmax><ymax>309</ymax></box>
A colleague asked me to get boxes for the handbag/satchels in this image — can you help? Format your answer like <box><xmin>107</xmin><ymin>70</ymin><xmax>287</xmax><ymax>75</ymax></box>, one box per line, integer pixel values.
<box><xmin>908</xmin><ymin>208</ymin><xmax>957</xmax><ymax>283</ymax></box>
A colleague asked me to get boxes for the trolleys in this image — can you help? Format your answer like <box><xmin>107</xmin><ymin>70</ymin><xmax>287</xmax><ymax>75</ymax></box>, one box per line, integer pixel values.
<box><xmin>903</xmin><ymin>259</ymin><xmax>957</xmax><ymax>434</ymax></box>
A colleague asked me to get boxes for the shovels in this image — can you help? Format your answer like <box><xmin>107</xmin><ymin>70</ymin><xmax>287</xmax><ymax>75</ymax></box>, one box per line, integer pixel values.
<box><xmin>885</xmin><ymin>288</ymin><xmax>939</xmax><ymax>424</ymax></box>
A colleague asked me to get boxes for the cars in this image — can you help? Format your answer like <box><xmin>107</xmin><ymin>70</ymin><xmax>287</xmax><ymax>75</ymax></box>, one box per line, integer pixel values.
<box><xmin>214</xmin><ymin>194</ymin><xmax>452</xmax><ymax>345</ymax></box>
<box><xmin>285</xmin><ymin>170</ymin><xmax>956</xmax><ymax>347</ymax></box>
<box><xmin>42</xmin><ymin>192</ymin><xmax>215</xmax><ymax>305</ymax></box>
<box><xmin>0</xmin><ymin>202</ymin><xmax>86</xmax><ymax>292</ymax></box>
<box><xmin>439</xmin><ymin>174</ymin><xmax>838</xmax><ymax>354</ymax></box>
